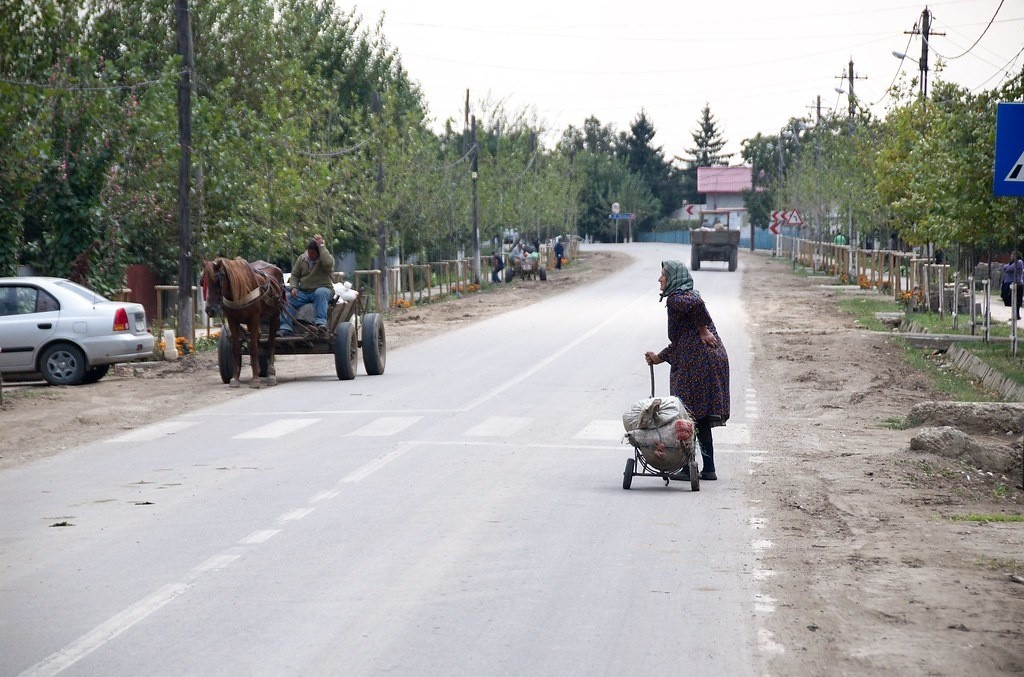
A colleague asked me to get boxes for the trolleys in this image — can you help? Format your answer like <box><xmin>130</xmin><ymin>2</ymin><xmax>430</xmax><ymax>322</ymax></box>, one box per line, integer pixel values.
<box><xmin>622</xmin><ymin>353</ymin><xmax>701</xmax><ymax>491</ymax></box>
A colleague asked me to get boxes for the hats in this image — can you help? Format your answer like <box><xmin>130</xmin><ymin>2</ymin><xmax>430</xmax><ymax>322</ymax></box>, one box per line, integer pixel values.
<box><xmin>306</xmin><ymin>239</ymin><xmax>318</xmax><ymax>250</ymax></box>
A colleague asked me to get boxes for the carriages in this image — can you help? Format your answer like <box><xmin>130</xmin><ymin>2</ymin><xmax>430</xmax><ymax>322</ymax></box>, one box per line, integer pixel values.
<box><xmin>199</xmin><ymin>257</ymin><xmax>387</xmax><ymax>388</ymax></box>
<box><xmin>506</xmin><ymin>235</ymin><xmax>546</xmax><ymax>282</ymax></box>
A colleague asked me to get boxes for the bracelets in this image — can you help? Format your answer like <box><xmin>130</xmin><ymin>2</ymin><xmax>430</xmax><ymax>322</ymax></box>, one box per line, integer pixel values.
<box><xmin>319</xmin><ymin>243</ymin><xmax>324</xmax><ymax>247</ymax></box>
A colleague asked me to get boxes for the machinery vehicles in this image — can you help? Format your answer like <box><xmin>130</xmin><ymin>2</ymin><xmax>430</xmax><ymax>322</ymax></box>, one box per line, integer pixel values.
<box><xmin>690</xmin><ymin>209</ymin><xmax>740</xmax><ymax>272</ymax></box>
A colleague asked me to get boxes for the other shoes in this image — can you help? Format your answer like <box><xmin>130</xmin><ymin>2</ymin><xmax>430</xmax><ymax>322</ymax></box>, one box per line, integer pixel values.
<box><xmin>277</xmin><ymin>330</ymin><xmax>291</xmax><ymax>336</ymax></box>
<box><xmin>700</xmin><ymin>471</ymin><xmax>717</xmax><ymax>480</ymax></box>
<box><xmin>319</xmin><ymin>325</ymin><xmax>328</xmax><ymax>331</ymax></box>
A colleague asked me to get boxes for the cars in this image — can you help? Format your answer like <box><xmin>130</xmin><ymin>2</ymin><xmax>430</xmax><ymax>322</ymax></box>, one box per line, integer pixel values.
<box><xmin>0</xmin><ymin>276</ymin><xmax>154</xmax><ymax>387</ymax></box>
<box><xmin>554</xmin><ymin>236</ymin><xmax>590</xmax><ymax>244</ymax></box>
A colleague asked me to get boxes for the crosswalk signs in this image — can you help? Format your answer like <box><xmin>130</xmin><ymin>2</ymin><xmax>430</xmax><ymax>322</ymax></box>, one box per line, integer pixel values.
<box><xmin>992</xmin><ymin>102</ymin><xmax>1024</xmax><ymax>197</ymax></box>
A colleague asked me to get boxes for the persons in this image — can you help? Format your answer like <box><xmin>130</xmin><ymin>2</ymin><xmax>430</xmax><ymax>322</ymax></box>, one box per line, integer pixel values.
<box><xmin>833</xmin><ymin>232</ymin><xmax>846</xmax><ymax>245</ymax></box>
<box><xmin>702</xmin><ymin>219</ymin><xmax>713</xmax><ymax>228</ymax></box>
<box><xmin>275</xmin><ymin>233</ymin><xmax>335</xmax><ymax>335</ymax></box>
<box><xmin>553</xmin><ymin>238</ymin><xmax>564</xmax><ymax>270</ymax></box>
<box><xmin>491</xmin><ymin>251</ymin><xmax>504</xmax><ymax>284</ymax></box>
<box><xmin>713</xmin><ymin>220</ymin><xmax>725</xmax><ymax>229</ymax></box>
<box><xmin>512</xmin><ymin>242</ymin><xmax>525</xmax><ymax>260</ymax></box>
<box><xmin>645</xmin><ymin>259</ymin><xmax>731</xmax><ymax>480</ymax></box>
<box><xmin>1000</xmin><ymin>251</ymin><xmax>1024</xmax><ymax>321</ymax></box>
